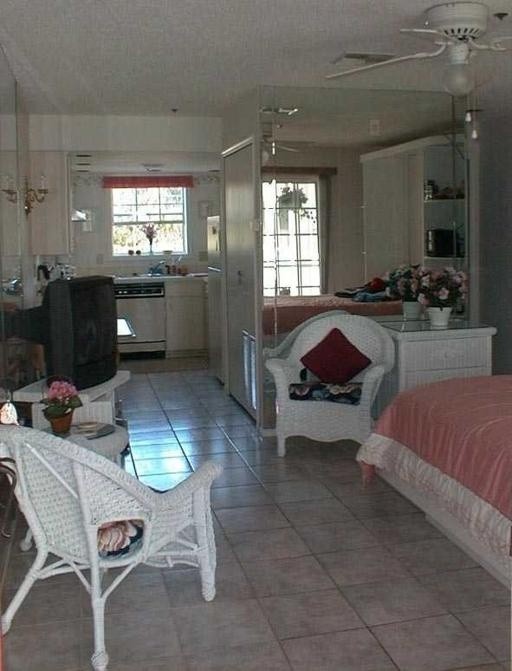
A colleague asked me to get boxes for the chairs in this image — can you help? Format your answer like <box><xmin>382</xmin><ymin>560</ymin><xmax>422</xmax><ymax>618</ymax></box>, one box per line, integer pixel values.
<box><xmin>265</xmin><ymin>310</ymin><xmax>395</xmax><ymax>458</ymax></box>
<box><xmin>0</xmin><ymin>424</ymin><xmax>224</xmax><ymax>671</ymax></box>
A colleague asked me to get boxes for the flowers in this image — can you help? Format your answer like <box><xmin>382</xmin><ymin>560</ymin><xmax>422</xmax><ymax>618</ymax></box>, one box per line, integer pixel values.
<box><xmin>385</xmin><ymin>263</ymin><xmax>422</xmax><ymax>298</ymax></box>
<box><xmin>141</xmin><ymin>224</ymin><xmax>157</xmax><ymax>244</ymax></box>
<box><xmin>39</xmin><ymin>380</ymin><xmax>82</xmax><ymax>418</ymax></box>
<box><xmin>419</xmin><ymin>267</ymin><xmax>471</xmax><ymax>315</ymax></box>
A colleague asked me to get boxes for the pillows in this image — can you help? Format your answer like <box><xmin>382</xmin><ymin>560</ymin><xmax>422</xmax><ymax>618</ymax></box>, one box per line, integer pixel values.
<box><xmin>299</xmin><ymin>327</ymin><xmax>371</xmax><ymax>390</ymax></box>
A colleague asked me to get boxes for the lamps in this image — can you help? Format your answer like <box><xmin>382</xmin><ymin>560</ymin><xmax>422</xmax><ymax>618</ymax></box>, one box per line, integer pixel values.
<box><xmin>24</xmin><ymin>173</ymin><xmax>48</xmax><ymax>216</ymax></box>
<box><xmin>461</xmin><ymin>57</ymin><xmax>487</xmax><ymax>140</ymax></box>
<box><xmin>1</xmin><ymin>172</ymin><xmax>17</xmax><ymax>204</ymax></box>
<box><xmin>423</xmin><ymin>61</ymin><xmax>489</xmax><ymax>96</ymax></box>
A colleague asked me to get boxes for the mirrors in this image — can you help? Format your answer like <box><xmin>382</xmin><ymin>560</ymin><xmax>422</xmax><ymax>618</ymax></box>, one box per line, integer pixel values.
<box><xmin>0</xmin><ymin>46</ymin><xmax>19</xmax><ymax>285</ymax></box>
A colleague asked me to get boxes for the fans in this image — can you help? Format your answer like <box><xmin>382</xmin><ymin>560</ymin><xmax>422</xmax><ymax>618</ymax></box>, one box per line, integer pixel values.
<box><xmin>326</xmin><ymin>26</ymin><xmax>512</xmax><ymax>85</ymax></box>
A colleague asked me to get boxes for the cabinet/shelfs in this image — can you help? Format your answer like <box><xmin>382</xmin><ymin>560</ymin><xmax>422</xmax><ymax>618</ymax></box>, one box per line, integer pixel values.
<box><xmin>358</xmin><ymin>132</ymin><xmax>481</xmax><ymax>321</ymax></box>
<box><xmin>26</xmin><ymin>149</ymin><xmax>75</xmax><ymax>256</ymax></box>
<box><xmin>166</xmin><ymin>279</ymin><xmax>207</xmax><ymax>357</ymax></box>
<box><xmin>12</xmin><ymin>371</ymin><xmax>130</xmax><ymax>425</ymax></box>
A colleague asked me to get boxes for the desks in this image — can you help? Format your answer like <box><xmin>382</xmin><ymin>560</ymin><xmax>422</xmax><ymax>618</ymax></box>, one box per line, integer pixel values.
<box><xmin>377</xmin><ymin>318</ymin><xmax>499</xmax><ymax>413</ymax></box>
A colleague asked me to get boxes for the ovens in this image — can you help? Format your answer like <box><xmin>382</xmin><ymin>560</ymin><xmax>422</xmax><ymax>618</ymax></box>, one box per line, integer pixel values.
<box><xmin>115</xmin><ymin>281</ymin><xmax>166</xmax><ymax>353</ymax></box>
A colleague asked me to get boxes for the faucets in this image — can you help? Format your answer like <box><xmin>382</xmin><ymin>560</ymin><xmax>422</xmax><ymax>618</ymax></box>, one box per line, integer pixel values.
<box><xmin>152</xmin><ymin>261</ymin><xmax>164</xmax><ymax>274</ymax></box>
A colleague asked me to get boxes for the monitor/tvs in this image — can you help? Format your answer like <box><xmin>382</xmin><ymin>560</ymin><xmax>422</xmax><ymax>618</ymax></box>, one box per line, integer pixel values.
<box><xmin>12</xmin><ymin>275</ymin><xmax>118</xmax><ymax>390</ymax></box>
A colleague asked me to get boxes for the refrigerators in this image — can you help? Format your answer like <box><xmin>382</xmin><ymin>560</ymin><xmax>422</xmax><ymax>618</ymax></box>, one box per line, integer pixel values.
<box><xmin>206</xmin><ymin>215</ymin><xmax>225</xmax><ymax>384</ymax></box>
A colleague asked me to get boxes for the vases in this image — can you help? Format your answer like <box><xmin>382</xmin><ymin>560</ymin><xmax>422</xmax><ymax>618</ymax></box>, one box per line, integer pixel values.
<box><xmin>427</xmin><ymin>306</ymin><xmax>452</xmax><ymax>329</ymax></box>
<box><xmin>45</xmin><ymin>410</ymin><xmax>75</xmax><ymax>435</ymax></box>
<box><xmin>149</xmin><ymin>240</ymin><xmax>153</xmax><ymax>253</ymax></box>
<box><xmin>404</xmin><ymin>301</ymin><xmax>421</xmax><ymax>322</ymax></box>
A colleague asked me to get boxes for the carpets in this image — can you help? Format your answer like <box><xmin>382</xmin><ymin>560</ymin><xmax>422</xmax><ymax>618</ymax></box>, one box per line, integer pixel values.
<box><xmin>131</xmin><ymin>359</ymin><xmax>209</xmax><ymax>374</ymax></box>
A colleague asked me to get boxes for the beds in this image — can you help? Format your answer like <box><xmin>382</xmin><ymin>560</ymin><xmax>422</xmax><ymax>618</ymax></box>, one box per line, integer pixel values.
<box><xmin>355</xmin><ymin>372</ymin><xmax>512</xmax><ymax>592</ymax></box>
